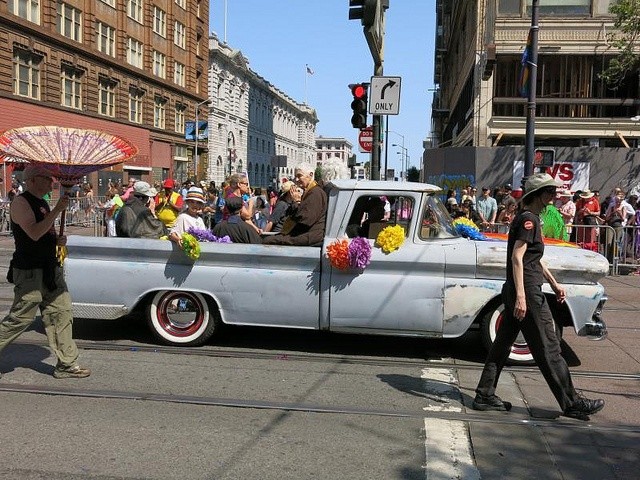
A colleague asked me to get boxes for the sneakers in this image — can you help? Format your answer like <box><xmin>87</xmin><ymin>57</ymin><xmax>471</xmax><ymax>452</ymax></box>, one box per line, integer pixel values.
<box><xmin>473</xmin><ymin>397</ymin><xmax>511</xmax><ymax>411</ymax></box>
<box><xmin>53</xmin><ymin>367</ymin><xmax>90</xmax><ymax>378</ymax></box>
<box><xmin>566</xmin><ymin>397</ymin><xmax>605</xmax><ymax>417</ymax></box>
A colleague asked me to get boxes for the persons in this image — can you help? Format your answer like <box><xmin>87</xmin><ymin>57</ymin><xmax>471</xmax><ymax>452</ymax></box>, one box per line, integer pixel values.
<box><xmin>626</xmin><ymin>194</ymin><xmax>640</xmax><ymax>258</ymax></box>
<box><xmin>261</xmin><ymin>161</ymin><xmax>328</xmax><ymax>246</ymax></box>
<box><xmin>202</xmin><ymin>188</ymin><xmax>219</xmax><ymax>230</ymax></box>
<box><xmin>212</xmin><ymin>193</ymin><xmax>262</xmax><ymax>244</ymax></box>
<box><xmin>249</xmin><ymin>188</ymin><xmax>256</xmax><ymax>198</ymax></box>
<box><xmin>179</xmin><ymin>182</ymin><xmax>187</xmax><ymax>195</ymax></box>
<box><xmin>146</xmin><ymin>188</ymin><xmax>158</xmax><ymax>216</ymax></box>
<box><xmin>445</xmin><ymin>188</ymin><xmax>457</xmax><ymax>208</ymax></box>
<box><xmin>461</xmin><ymin>185</ymin><xmax>472</xmax><ymax>204</ymax></box>
<box><xmin>447</xmin><ymin>197</ymin><xmax>458</xmax><ymax>213</ymax></box>
<box><xmin>467</xmin><ymin>186</ymin><xmax>477</xmax><ymax>211</ymax></box>
<box><xmin>106</xmin><ymin>181</ymin><xmax>114</xmax><ymax>197</ymax></box>
<box><xmin>242</xmin><ymin>188</ymin><xmax>250</xmax><ymax>202</ymax></box>
<box><xmin>8</xmin><ymin>182</ymin><xmax>20</xmax><ymax>231</ymax></box>
<box><xmin>361</xmin><ymin>196</ymin><xmax>386</xmax><ymax>238</ymax></box>
<box><xmin>181</xmin><ymin>179</ymin><xmax>195</xmax><ymax>209</ymax></box>
<box><xmin>115</xmin><ymin>180</ymin><xmax>169</xmax><ymax>238</ymax></box>
<box><xmin>227</xmin><ymin>176</ymin><xmax>239</xmax><ymax>192</ymax></box>
<box><xmin>153</xmin><ymin>180</ymin><xmax>161</xmax><ymax>199</ymax></box>
<box><xmin>573</xmin><ymin>190</ymin><xmax>582</xmax><ymax>202</ymax></box>
<box><xmin>73</xmin><ymin>184</ymin><xmax>81</xmax><ymax>224</ymax></box>
<box><xmin>397</xmin><ymin>199</ymin><xmax>413</xmax><ymax>222</ymax></box>
<box><xmin>97</xmin><ymin>181</ymin><xmax>123</xmax><ymax>237</ymax></box>
<box><xmin>591</xmin><ymin>191</ymin><xmax>600</xmax><ymax>201</ymax></box>
<box><xmin>83</xmin><ymin>185</ymin><xmax>93</xmax><ymax>226</ymax></box>
<box><xmin>154</xmin><ymin>179</ymin><xmax>184</xmax><ymax>240</ymax></box>
<box><xmin>422</xmin><ymin>204</ymin><xmax>439</xmax><ymax>225</ymax></box>
<box><xmin>380</xmin><ymin>196</ymin><xmax>392</xmax><ymax>222</ymax></box>
<box><xmin>601</xmin><ymin>196</ymin><xmax>611</xmax><ymax>245</ymax></box>
<box><xmin>494</xmin><ymin>183</ymin><xmax>516</xmax><ymax>233</ymax></box>
<box><xmin>222</xmin><ymin>173</ymin><xmax>263</xmax><ymax>236</ymax></box>
<box><xmin>0</xmin><ymin>164</ymin><xmax>91</xmax><ymax>379</ymax></box>
<box><xmin>154</xmin><ymin>181</ymin><xmax>166</xmax><ymax>207</ymax></box>
<box><xmin>492</xmin><ymin>186</ymin><xmax>502</xmax><ymax>199</ymax></box>
<box><xmin>266</xmin><ymin>186</ymin><xmax>275</xmax><ymax>205</ymax></box>
<box><xmin>87</xmin><ymin>184</ymin><xmax>95</xmax><ymax>197</ymax></box>
<box><xmin>496</xmin><ymin>198</ymin><xmax>516</xmax><ymax>233</ymax></box>
<box><xmin>613</xmin><ymin>187</ymin><xmax>621</xmax><ymax>195</ymax></box>
<box><xmin>209</xmin><ymin>181</ymin><xmax>219</xmax><ymax>197</ymax></box>
<box><xmin>558</xmin><ymin>189</ymin><xmax>576</xmax><ymax>242</ymax></box>
<box><xmin>455</xmin><ymin>199</ymin><xmax>483</xmax><ymax>227</ymax></box>
<box><xmin>576</xmin><ymin>189</ymin><xmax>601</xmax><ymax>243</ymax></box>
<box><xmin>220</xmin><ymin>182</ymin><xmax>228</xmax><ymax>197</ymax></box>
<box><xmin>605</xmin><ymin>195</ymin><xmax>626</xmax><ymax>264</ymax></box>
<box><xmin>617</xmin><ymin>190</ymin><xmax>636</xmax><ymax>228</ymax></box>
<box><xmin>200</xmin><ymin>180</ymin><xmax>206</xmax><ymax>190</ymax></box>
<box><xmin>120</xmin><ymin>177</ymin><xmax>140</xmax><ymax>202</ymax></box>
<box><xmin>263</xmin><ymin>181</ymin><xmax>297</xmax><ymax>232</ymax></box>
<box><xmin>167</xmin><ymin>186</ymin><xmax>208</xmax><ymax>251</ymax></box>
<box><xmin>121</xmin><ymin>184</ymin><xmax>127</xmax><ymax>195</ymax></box>
<box><xmin>459</xmin><ymin>189</ymin><xmax>467</xmax><ymax>208</ymax></box>
<box><xmin>252</xmin><ymin>187</ymin><xmax>266</xmax><ymax>208</ymax></box>
<box><xmin>255</xmin><ymin>197</ymin><xmax>270</xmax><ymax>219</ymax></box>
<box><xmin>476</xmin><ymin>185</ymin><xmax>498</xmax><ymax>229</ymax></box>
<box><xmin>175</xmin><ymin>299</ymin><xmax>182</xmax><ymax>315</ymax></box>
<box><xmin>472</xmin><ymin>172</ymin><xmax>605</xmax><ymax>418</ymax></box>
<box><xmin>267</xmin><ymin>191</ymin><xmax>279</xmax><ymax>216</ymax></box>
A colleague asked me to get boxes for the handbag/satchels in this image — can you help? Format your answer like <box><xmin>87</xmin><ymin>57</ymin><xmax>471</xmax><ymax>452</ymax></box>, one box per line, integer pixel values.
<box><xmin>606</xmin><ymin>212</ymin><xmax>624</xmax><ymax>225</ymax></box>
<box><xmin>584</xmin><ymin>215</ymin><xmax>596</xmax><ymax>225</ymax></box>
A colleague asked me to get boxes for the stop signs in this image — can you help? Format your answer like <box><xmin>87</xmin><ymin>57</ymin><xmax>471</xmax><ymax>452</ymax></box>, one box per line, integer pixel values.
<box><xmin>358</xmin><ymin>126</ymin><xmax>372</xmax><ymax>152</ymax></box>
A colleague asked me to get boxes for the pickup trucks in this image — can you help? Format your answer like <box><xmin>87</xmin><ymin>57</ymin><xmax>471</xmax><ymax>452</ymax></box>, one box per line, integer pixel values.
<box><xmin>58</xmin><ymin>176</ymin><xmax>613</xmax><ymax>365</ymax></box>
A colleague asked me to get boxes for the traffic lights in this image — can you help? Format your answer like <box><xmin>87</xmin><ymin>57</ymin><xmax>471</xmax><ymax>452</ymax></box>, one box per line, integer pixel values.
<box><xmin>350</xmin><ymin>83</ymin><xmax>367</xmax><ymax>129</ymax></box>
<box><xmin>534</xmin><ymin>149</ymin><xmax>554</xmax><ymax>167</ymax></box>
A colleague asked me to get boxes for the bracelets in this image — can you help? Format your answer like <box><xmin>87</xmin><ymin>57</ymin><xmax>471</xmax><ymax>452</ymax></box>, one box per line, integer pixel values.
<box><xmin>292</xmin><ymin>198</ymin><xmax>302</xmax><ymax>204</ymax></box>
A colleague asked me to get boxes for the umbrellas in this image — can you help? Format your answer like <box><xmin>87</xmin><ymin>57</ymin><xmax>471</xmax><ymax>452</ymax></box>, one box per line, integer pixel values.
<box><xmin>0</xmin><ymin>125</ymin><xmax>140</xmax><ymax>267</ymax></box>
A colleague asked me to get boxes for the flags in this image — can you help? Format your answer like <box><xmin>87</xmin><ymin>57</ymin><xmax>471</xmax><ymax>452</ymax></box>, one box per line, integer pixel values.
<box><xmin>518</xmin><ymin>27</ymin><xmax>532</xmax><ymax>98</ymax></box>
<box><xmin>307</xmin><ymin>67</ymin><xmax>314</xmax><ymax>75</ymax></box>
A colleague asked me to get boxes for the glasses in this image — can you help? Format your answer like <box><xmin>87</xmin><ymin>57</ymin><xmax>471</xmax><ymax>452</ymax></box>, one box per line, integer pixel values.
<box><xmin>36</xmin><ymin>175</ymin><xmax>52</xmax><ymax>180</ymax></box>
<box><xmin>239</xmin><ymin>183</ymin><xmax>248</xmax><ymax>187</ymax></box>
<box><xmin>482</xmin><ymin>189</ymin><xmax>488</xmax><ymax>191</ymax></box>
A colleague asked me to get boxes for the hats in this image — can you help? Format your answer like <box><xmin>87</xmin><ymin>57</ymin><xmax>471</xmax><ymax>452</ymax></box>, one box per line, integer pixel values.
<box><xmin>579</xmin><ymin>191</ymin><xmax>595</xmax><ymax>198</ymax></box>
<box><xmin>505</xmin><ymin>186</ymin><xmax>512</xmax><ymax>190</ymax></box>
<box><xmin>520</xmin><ymin>174</ymin><xmax>557</xmax><ymax>199</ymax></box>
<box><xmin>449</xmin><ymin>198</ymin><xmax>457</xmax><ymax>205</ymax></box>
<box><xmin>365</xmin><ymin>198</ymin><xmax>386</xmax><ymax>208</ymax></box>
<box><xmin>134</xmin><ymin>183</ymin><xmax>157</xmax><ymax>196</ymax></box>
<box><xmin>561</xmin><ymin>190</ymin><xmax>573</xmax><ymax>197</ymax></box>
<box><xmin>163</xmin><ymin>180</ymin><xmax>174</xmax><ymax>188</ymax></box>
<box><xmin>184</xmin><ymin>187</ymin><xmax>204</xmax><ymax>203</ymax></box>
<box><xmin>227</xmin><ymin>198</ymin><xmax>242</xmax><ymax>213</ymax></box>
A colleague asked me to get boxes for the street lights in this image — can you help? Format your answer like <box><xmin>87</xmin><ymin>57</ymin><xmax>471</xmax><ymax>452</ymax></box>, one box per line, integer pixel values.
<box><xmin>194</xmin><ymin>97</ymin><xmax>215</xmax><ymax>183</ymax></box>
